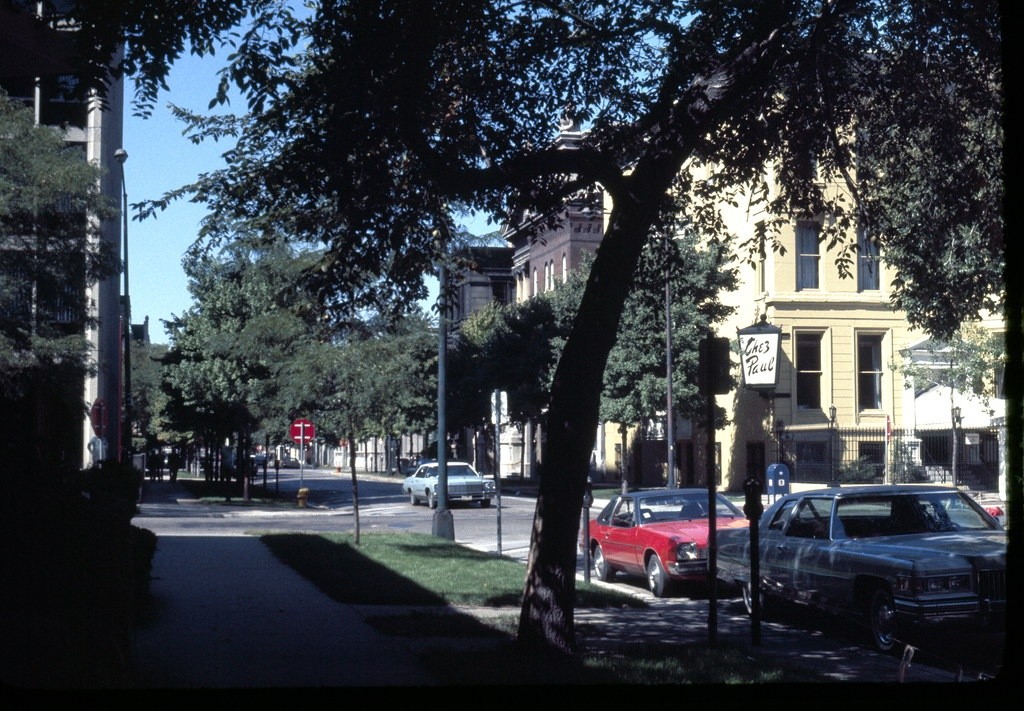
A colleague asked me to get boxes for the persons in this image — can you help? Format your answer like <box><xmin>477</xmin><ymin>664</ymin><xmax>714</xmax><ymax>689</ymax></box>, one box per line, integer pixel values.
<box><xmin>157</xmin><ymin>447</ymin><xmax>166</xmax><ymax>482</ymax></box>
<box><xmin>167</xmin><ymin>448</ymin><xmax>181</xmax><ymax>483</ymax></box>
<box><xmin>149</xmin><ymin>450</ymin><xmax>158</xmax><ymax>482</ymax></box>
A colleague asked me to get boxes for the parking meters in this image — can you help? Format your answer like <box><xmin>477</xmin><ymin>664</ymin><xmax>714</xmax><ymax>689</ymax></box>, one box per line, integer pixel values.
<box><xmin>743</xmin><ymin>477</ymin><xmax>764</xmax><ymax>658</ymax></box>
<box><xmin>582</xmin><ymin>476</ymin><xmax>593</xmax><ymax>583</ymax></box>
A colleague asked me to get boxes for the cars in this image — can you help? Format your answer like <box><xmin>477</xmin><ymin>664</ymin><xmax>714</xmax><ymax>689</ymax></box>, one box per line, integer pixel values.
<box><xmin>402</xmin><ymin>457</ymin><xmax>497</xmax><ymax>509</ymax></box>
<box><xmin>579</xmin><ymin>488</ymin><xmax>750</xmax><ymax>598</ymax></box>
<box><xmin>707</xmin><ymin>485</ymin><xmax>1008</xmax><ymax>655</ymax></box>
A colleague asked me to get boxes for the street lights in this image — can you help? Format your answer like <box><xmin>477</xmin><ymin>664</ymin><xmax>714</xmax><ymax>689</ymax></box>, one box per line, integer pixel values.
<box><xmin>827</xmin><ymin>405</ymin><xmax>839</xmax><ymax>487</ymax></box>
<box><xmin>114</xmin><ymin>149</ymin><xmax>131</xmax><ymax>418</ymax></box>
<box><xmin>953</xmin><ymin>406</ymin><xmax>965</xmax><ymax>482</ymax></box>
